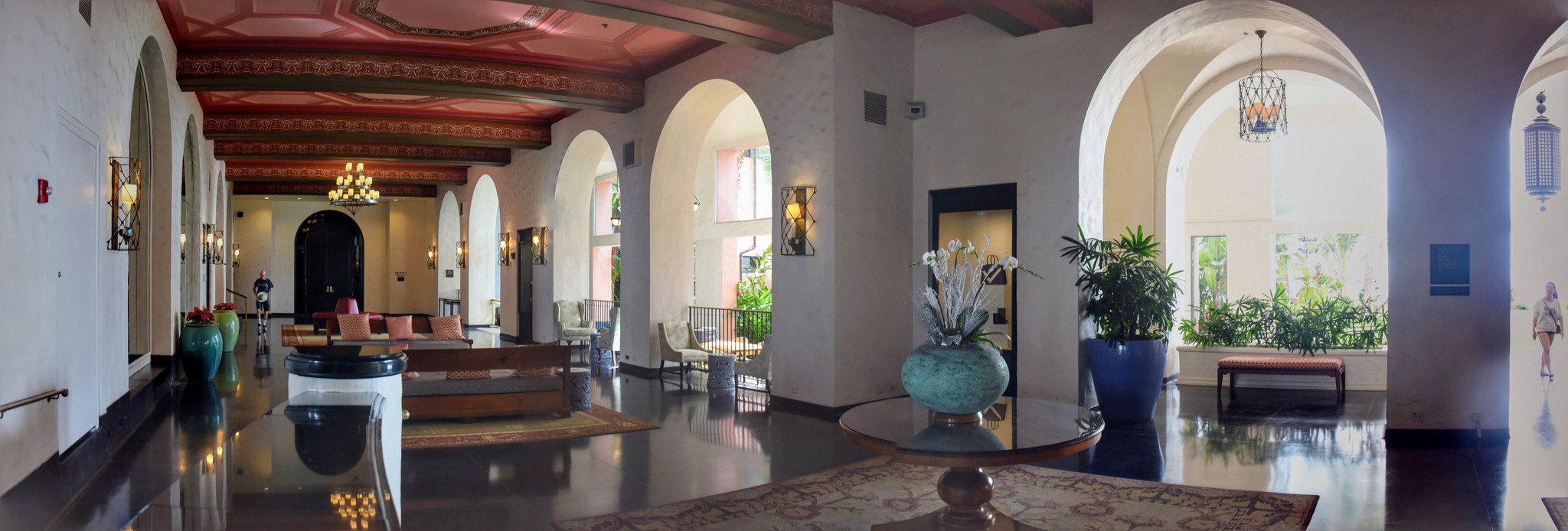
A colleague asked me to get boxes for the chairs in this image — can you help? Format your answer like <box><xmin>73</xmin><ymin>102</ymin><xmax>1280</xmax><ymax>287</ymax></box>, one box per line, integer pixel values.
<box><xmin>553</xmin><ymin>300</ymin><xmax>773</xmax><ymax>396</ymax></box>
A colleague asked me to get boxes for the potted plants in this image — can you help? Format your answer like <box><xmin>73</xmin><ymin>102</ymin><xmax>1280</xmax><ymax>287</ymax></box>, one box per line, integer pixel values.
<box><xmin>1059</xmin><ymin>223</ymin><xmax>1183</xmax><ymax>425</ymax></box>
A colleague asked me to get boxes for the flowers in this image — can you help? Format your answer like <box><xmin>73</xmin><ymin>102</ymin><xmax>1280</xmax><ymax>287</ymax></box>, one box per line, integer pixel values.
<box><xmin>909</xmin><ymin>234</ymin><xmax>1043</xmax><ymax>356</ymax></box>
<box><xmin>185</xmin><ymin>302</ymin><xmax>237</xmax><ymax>323</ymax></box>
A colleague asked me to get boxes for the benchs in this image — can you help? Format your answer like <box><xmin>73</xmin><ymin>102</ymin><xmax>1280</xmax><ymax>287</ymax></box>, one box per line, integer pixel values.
<box><xmin>396</xmin><ymin>343</ymin><xmax>572</xmax><ymax>420</ymax></box>
<box><xmin>326</xmin><ymin>313</ymin><xmax>473</xmax><ymax>349</ymax></box>
<box><xmin>1217</xmin><ymin>354</ymin><xmax>1346</xmax><ymax>406</ymax></box>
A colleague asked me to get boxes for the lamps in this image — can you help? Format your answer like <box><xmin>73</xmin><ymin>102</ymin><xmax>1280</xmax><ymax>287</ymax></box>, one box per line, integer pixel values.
<box><xmin>107</xmin><ymin>156</ymin><xmax>240</xmax><ymax>268</ymax></box>
<box><xmin>611</xmin><ymin>170</ymin><xmax>816</xmax><ymax>256</ymax></box>
<box><xmin>1524</xmin><ymin>89</ymin><xmax>1563</xmax><ymax>212</ymax></box>
<box><xmin>329</xmin><ymin>160</ymin><xmax>380</xmax><ymax>216</ymax></box>
<box><xmin>1238</xmin><ymin>33</ymin><xmax>1288</xmax><ymax>141</ymax></box>
<box><xmin>427</xmin><ymin>226</ymin><xmax>547</xmax><ymax>269</ymax></box>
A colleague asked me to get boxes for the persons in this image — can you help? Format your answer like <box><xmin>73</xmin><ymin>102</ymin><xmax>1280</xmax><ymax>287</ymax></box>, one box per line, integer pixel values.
<box><xmin>253</xmin><ymin>270</ymin><xmax>274</xmax><ymax>330</ymax></box>
<box><xmin>1532</xmin><ymin>282</ymin><xmax>1564</xmax><ymax>379</ymax></box>
<box><xmin>253</xmin><ymin>330</ymin><xmax>272</xmax><ymax>388</ymax></box>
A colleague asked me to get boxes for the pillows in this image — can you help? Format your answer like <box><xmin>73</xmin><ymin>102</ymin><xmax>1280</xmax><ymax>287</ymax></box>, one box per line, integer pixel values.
<box><xmin>389</xmin><ymin>341</ymin><xmax>559</xmax><ymax>377</ymax></box>
<box><xmin>337</xmin><ymin>313</ymin><xmax>466</xmax><ymax>340</ymax></box>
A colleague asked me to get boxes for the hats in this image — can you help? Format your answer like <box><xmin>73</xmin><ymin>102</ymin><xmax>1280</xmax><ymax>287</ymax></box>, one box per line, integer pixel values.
<box><xmin>256</xmin><ymin>292</ymin><xmax>268</xmax><ymax>302</ymax></box>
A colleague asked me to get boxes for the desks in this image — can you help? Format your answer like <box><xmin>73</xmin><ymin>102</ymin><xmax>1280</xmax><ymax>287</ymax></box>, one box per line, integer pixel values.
<box><xmin>701</xmin><ymin>339</ymin><xmax>763</xmax><ymax>360</ymax></box>
<box><xmin>838</xmin><ymin>393</ymin><xmax>1106</xmax><ymax>531</ymax></box>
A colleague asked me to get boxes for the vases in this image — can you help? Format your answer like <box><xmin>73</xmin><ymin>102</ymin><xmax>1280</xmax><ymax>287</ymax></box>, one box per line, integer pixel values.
<box><xmin>901</xmin><ymin>341</ymin><xmax>1009</xmax><ymax>422</ymax></box>
<box><xmin>178</xmin><ymin>310</ymin><xmax>239</xmax><ymax>383</ymax></box>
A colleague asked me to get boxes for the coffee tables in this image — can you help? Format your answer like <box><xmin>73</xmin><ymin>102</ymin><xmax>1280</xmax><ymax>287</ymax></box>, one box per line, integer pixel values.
<box><xmin>558</xmin><ymin>367</ymin><xmax>591</xmax><ymax>410</ymax></box>
<box><xmin>708</xmin><ymin>353</ymin><xmax>737</xmax><ymax>389</ymax></box>
<box><xmin>590</xmin><ymin>334</ymin><xmax>612</xmax><ymax>359</ymax></box>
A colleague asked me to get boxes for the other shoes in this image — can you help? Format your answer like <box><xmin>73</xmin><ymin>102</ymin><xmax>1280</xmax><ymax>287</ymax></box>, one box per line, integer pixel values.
<box><xmin>257</xmin><ymin>323</ymin><xmax>262</xmax><ymax>331</ymax></box>
<box><xmin>262</xmin><ymin>325</ymin><xmax>267</xmax><ymax>329</ymax></box>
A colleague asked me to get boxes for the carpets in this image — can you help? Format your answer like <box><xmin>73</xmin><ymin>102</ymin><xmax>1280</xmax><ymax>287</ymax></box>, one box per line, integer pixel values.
<box><xmin>551</xmin><ymin>463</ymin><xmax>1321</xmax><ymax>531</ymax></box>
<box><xmin>402</xmin><ymin>401</ymin><xmax>662</xmax><ymax>452</ymax></box>
<box><xmin>281</xmin><ymin>323</ymin><xmax>341</xmax><ymax>347</ymax></box>
